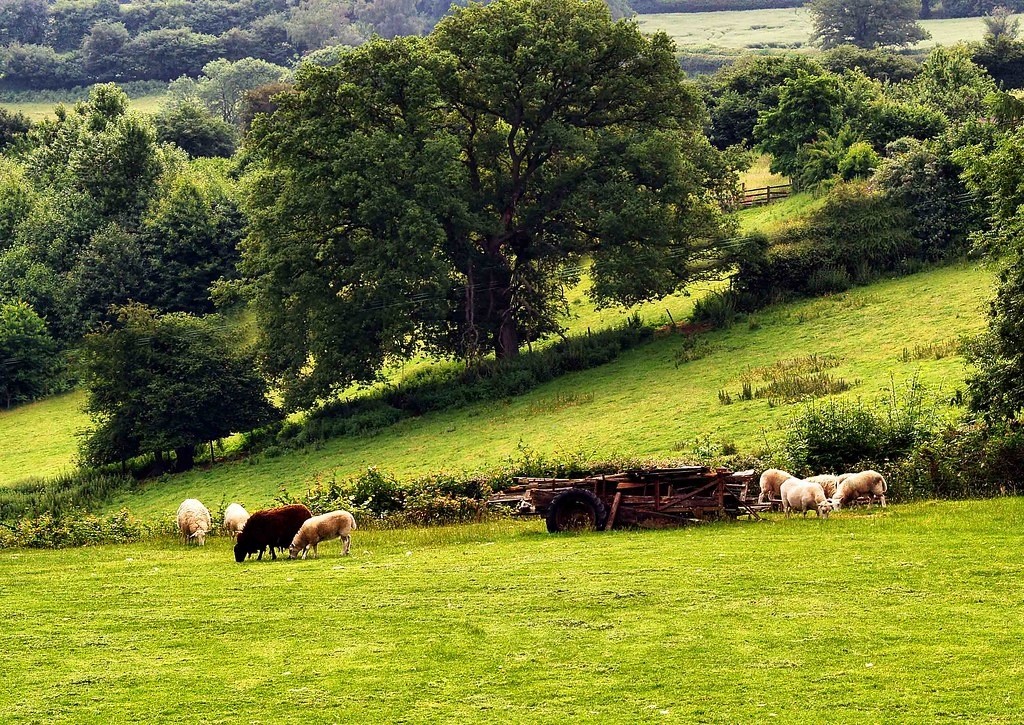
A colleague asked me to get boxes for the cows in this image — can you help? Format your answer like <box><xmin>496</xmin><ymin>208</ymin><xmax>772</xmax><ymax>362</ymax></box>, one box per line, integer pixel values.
<box><xmin>176</xmin><ymin>499</ymin><xmax>212</xmax><ymax>547</ymax></box>
<box><xmin>758</xmin><ymin>468</ymin><xmax>888</xmax><ymax>520</ymax></box>
<box><xmin>223</xmin><ymin>503</ymin><xmax>357</xmax><ymax>562</ymax></box>
